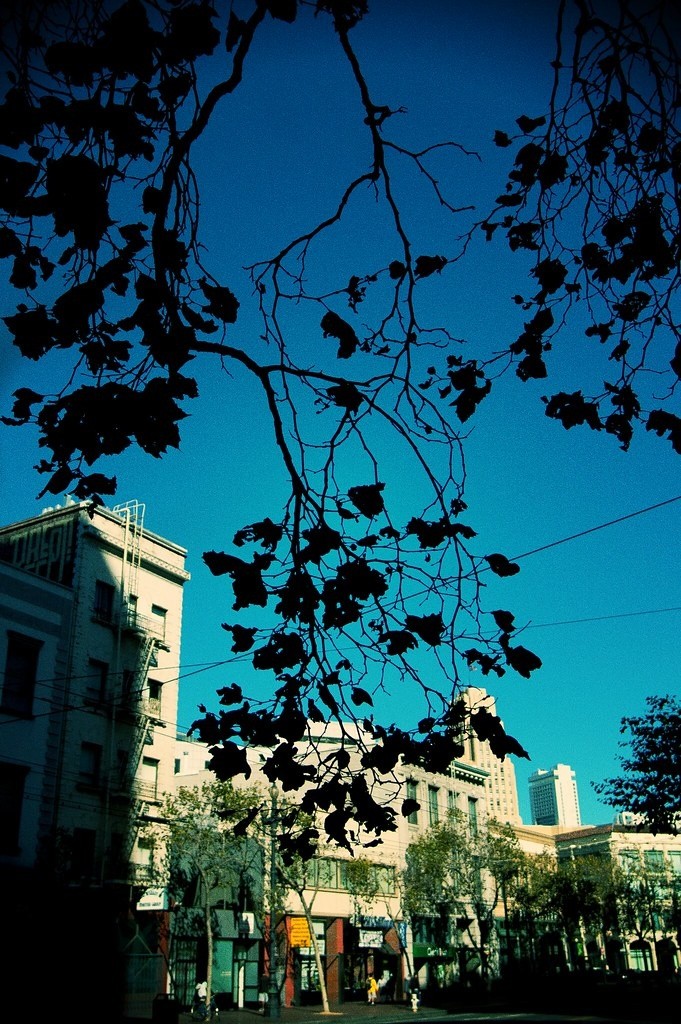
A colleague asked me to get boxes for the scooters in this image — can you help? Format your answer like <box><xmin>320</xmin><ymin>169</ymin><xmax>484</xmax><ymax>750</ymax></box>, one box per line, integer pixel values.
<box><xmin>192</xmin><ymin>991</ymin><xmax>219</xmax><ymax>1020</ymax></box>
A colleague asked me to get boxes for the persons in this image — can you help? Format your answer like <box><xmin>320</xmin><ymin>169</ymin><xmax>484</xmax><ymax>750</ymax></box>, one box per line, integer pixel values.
<box><xmin>365</xmin><ymin>973</ymin><xmax>387</xmax><ymax>1005</ymax></box>
<box><xmin>195</xmin><ymin>975</ymin><xmax>219</xmax><ymax>1015</ymax></box>
<box><xmin>408</xmin><ymin>971</ymin><xmax>422</xmax><ymax>1006</ymax></box>
